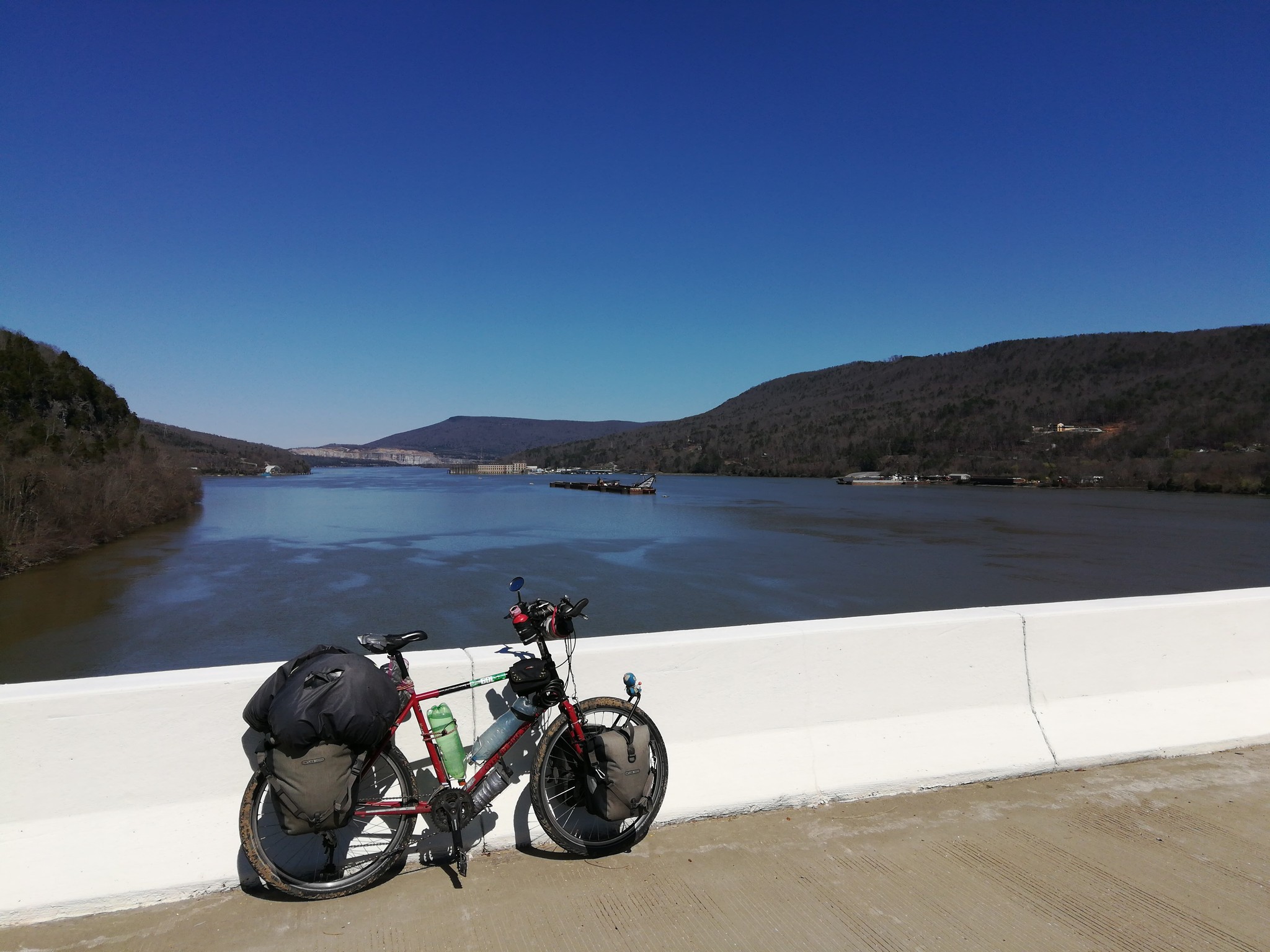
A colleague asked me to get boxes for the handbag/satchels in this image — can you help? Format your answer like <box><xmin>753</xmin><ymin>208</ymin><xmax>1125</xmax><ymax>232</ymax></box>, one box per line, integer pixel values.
<box><xmin>253</xmin><ymin>738</ymin><xmax>360</xmax><ymax>851</ymax></box>
<box><xmin>242</xmin><ymin>644</ymin><xmax>399</xmax><ymax>759</ymax></box>
<box><xmin>581</xmin><ymin>724</ymin><xmax>654</xmax><ymax>819</ymax></box>
<box><xmin>554</xmin><ymin>720</ymin><xmax>608</xmax><ymax>809</ymax></box>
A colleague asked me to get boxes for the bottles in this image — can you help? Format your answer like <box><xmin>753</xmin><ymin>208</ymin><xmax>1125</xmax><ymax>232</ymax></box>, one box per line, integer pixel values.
<box><xmin>427</xmin><ymin>703</ymin><xmax>467</xmax><ymax>786</ymax></box>
<box><xmin>467</xmin><ymin>696</ymin><xmax>538</xmax><ymax>765</ymax></box>
<box><xmin>472</xmin><ymin>766</ymin><xmax>514</xmax><ymax>814</ymax></box>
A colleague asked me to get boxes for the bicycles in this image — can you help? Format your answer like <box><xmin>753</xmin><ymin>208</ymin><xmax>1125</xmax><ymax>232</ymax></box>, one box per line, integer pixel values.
<box><xmin>238</xmin><ymin>576</ymin><xmax>668</xmax><ymax>901</ymax></box>
